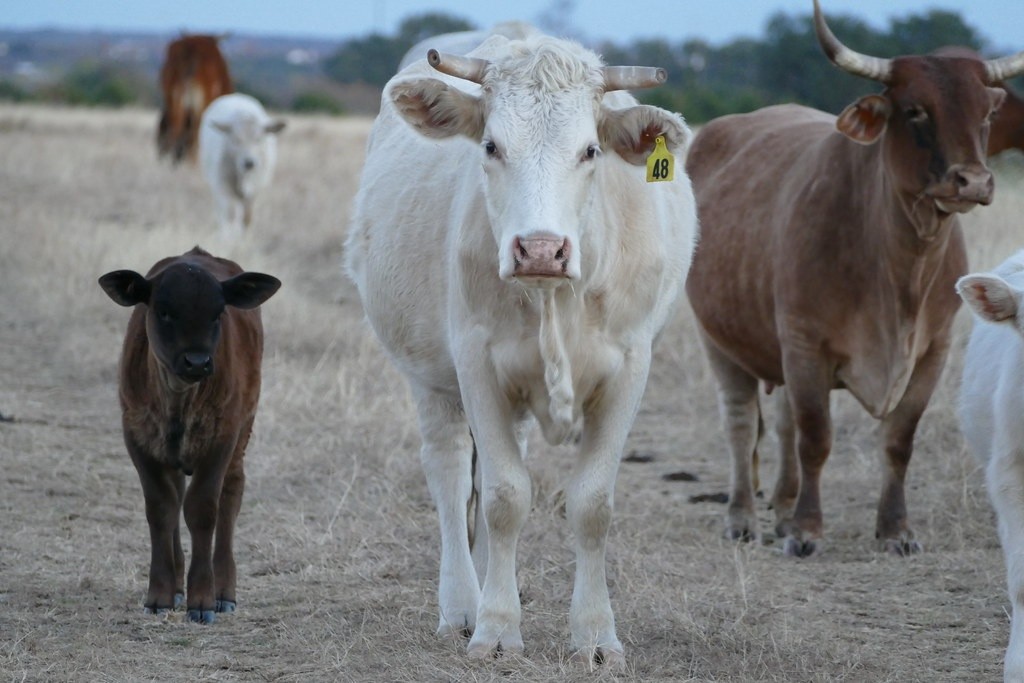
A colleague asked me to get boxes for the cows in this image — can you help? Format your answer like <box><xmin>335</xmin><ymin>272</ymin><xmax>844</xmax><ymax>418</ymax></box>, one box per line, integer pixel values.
<box><xmin>154</xmin><ymin>27</ymin><xmax>288</xmax><ymax>238</ymax></box>
<box><xmin>339</xmin><ymin>0</ymin><xmax>1024</xmax><ymax>683</ymax></box>
<box><xmin>96</xmin><ymin>244</ymin><xmax>283</xmax><ymax>625</ymax></box>
